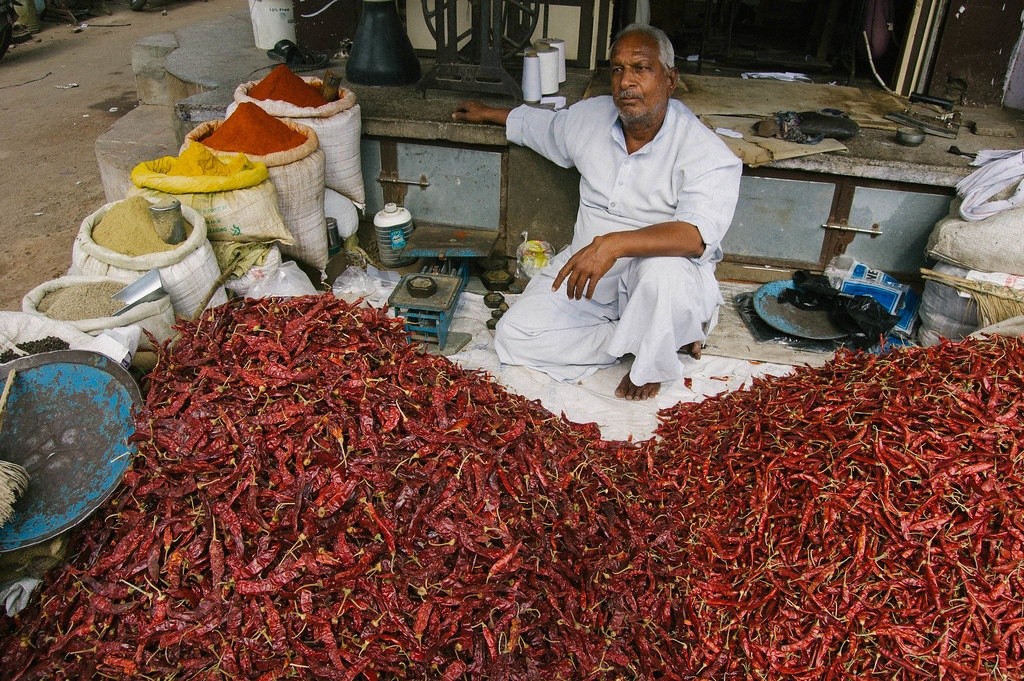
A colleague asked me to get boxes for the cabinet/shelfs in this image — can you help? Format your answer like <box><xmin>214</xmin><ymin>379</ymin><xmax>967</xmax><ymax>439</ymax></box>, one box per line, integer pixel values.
<box><xmin>171</xmin><ymin>100</ymin><xmax>509</xmax><ymax>242</ymax></box>
<box><xmin>714</xmin><ymin>149</ymin><xmax>967</xmax><ymax>286</ymax></box>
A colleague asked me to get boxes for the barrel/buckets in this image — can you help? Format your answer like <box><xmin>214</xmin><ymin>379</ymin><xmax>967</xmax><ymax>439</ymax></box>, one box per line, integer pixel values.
<box><xmin>248</xmin><ymin>0</ymin><xmax>297</xmax><ymax>49</ymax></box>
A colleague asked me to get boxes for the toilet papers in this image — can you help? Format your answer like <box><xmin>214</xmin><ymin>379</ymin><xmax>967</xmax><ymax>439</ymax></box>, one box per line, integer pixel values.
<box><xmin>535</xmin><ymin>37</ymin><xmax>567</xmax><ymax>84</ymax></box>
<box><xmin>522</xmin><ymin>46</ymin><xmax>561</xmax><ymax>96</ymax></box>
<box><xmin>520</xmin><ymin>55</ymin><xmax>543</xmax><ymax>104</ymax></box>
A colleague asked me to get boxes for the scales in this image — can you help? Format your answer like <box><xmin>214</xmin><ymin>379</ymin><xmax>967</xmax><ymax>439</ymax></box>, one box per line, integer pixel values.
<box><xmin>387</xmin><ymin>225</ymin><xmax>500</xmax><ymax>349</ymax></box>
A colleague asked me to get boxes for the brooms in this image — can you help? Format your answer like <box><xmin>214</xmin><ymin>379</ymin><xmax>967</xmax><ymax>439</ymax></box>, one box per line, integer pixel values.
<box><xmin>0</xmin><ymin>367</ymin><xmax>35</xmax><ymax>536</ymax></box>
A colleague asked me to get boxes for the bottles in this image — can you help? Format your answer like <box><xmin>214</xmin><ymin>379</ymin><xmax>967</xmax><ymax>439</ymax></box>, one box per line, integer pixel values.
<box><xmin>373</xmin><ymin>202</ymin><xmax>416</xmax><ymax>268</ymax></box>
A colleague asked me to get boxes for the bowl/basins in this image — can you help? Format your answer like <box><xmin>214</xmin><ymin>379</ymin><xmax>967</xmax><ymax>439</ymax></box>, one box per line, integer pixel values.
<box><xmin>896</xmin><ymin>126</ymin><xmax>926</xmax><ymax>146</ymax></box>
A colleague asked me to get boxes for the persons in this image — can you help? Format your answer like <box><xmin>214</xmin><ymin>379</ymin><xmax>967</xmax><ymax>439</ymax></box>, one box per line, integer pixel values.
<box><xmin>449</xmin><ymin>22</ymin><xmax>743</xmax><ymax>399</ymax></box>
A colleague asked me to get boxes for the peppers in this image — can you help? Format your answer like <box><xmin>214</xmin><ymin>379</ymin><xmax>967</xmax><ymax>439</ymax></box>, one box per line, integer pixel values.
<box><xmin>0</xmin><ymin>292</ymin><xmax>1024</xmax><ymax>681</ymax></box>
